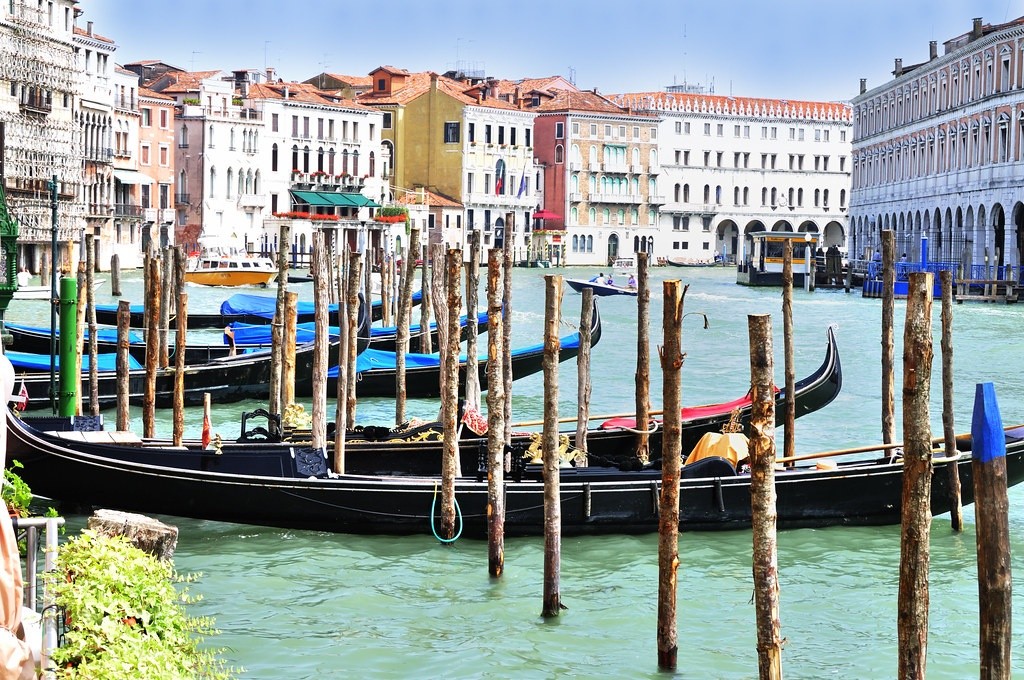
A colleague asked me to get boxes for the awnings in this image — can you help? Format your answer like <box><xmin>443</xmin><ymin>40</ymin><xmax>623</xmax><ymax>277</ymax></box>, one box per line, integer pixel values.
<box><xmin>287</xmin><ymin>189</ymin><xmax>382</xmax><ymax>208</ymax></box>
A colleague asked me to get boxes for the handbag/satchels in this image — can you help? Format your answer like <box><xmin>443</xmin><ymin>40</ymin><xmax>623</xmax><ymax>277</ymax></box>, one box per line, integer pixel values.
<box><xmin>288</xmin><ymin>447</ymin><xmax>328</xmax><ymax>478</ymax></box>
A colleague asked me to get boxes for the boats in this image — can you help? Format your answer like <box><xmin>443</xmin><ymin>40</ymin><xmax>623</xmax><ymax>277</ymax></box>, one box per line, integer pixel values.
<box><xmin>1</xmin><ymin>290</ymin><xmax>505</xmax><ymax>368</ymax></box>
<box><xmin>565</xmin><ymin>278</ymin><xmax>638</xmax><ymax>297</ymax></box>
<box><xmin>5</xmin><ymin>405</ymin><xmax>1024</xmax><ymax>537</ymax></box>
<box><xmin>183</xmin><ymin>237</ymin><xmax>280</xmax><ymax>288</ymax></box>
<box><xmin>21</xmin><ymin>326</ymin><xmax>842</xmax><ymax>476</ymax></box>
<box><xmin>3</xmin><ymin>295</ymin><xmax>602</xmax><ymax>412</ymax></box>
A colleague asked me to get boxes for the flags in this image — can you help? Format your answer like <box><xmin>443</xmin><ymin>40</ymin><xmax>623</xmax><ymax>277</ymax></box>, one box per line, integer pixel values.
<box><xmin>495</xmin><ymin>162</ymin><xmax>504</xmax><ymax>197</ymax></box>
<box><xmin>516</xmin><ymin>167</ymin><xmax>526</xmax><ymax>199</ymax></box>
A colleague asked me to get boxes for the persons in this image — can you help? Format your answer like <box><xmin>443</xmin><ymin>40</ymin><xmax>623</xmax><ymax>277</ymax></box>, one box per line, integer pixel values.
<box><xmin>875</xmin><ymin>249</ymin><xmax>881</xmax><ymax>271</ymax></box>
<box><xmin>17</xmin><ymin>267</ymin><xmax>32</xmax><ymax>286</ymax></box>
<box><xmin>901</xmin><ymin>253</ymin><xmax>907</xmax><ymax>276</ymax></box>
<box><xmin>607</xmin><ymin>275</ymin><xmax>613</xmax><ymax>286</ymax></box>
<box><xmin>628</xmin><ymin>275</ymin><xmax>636</xmax><ymax>288</ymax></box>
<box><xmin>713</xmin><ymin>249</ymin><xmax>721</xmax><ymax>262</ymax></box>
<box><xmin>596</xmin><ymin>273</ymin><xmax>607</xmax><ymax>285</ymax></box>
